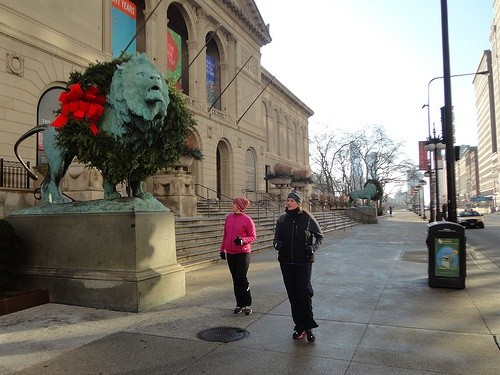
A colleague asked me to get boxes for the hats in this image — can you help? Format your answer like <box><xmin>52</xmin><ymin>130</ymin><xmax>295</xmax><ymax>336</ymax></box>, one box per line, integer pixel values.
<box><xmin>288</xmin><ymin>190</ymin><xmax>303</xmax><ymax>203</ymax></box>
<box><xmin>232</xmin><ymin>197</ymin><xmax>250</xmax><ymax>212</ymax></box>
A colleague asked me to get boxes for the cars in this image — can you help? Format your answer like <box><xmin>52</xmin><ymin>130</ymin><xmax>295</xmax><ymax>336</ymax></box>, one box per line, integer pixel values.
<box><xmin>457</xmin><ymin>207</ymin><xmax>497</xmax><ymax>217</ymax></box>
<box><xmin>415</xmin><ymin>208</ymin><xmax>431</xmax><ymax>219</ymax></box>
<box><xmin>457</xmin><ymin>210</ymin><xmax>485</xmax><ymax>229</ymax></box>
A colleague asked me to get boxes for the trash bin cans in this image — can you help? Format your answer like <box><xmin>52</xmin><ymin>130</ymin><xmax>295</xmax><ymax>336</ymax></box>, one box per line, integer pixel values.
<box><xmin>426</xmin><ymin>220</ymin><xmax>467</xmax><ymax>289</ymax></box>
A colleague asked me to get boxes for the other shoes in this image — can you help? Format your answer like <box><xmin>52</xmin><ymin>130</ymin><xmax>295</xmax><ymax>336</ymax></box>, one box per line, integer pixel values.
<box><xmin>245</xmin><ymin>305</ymin><xmax>252</xmax><ymax>315</ymax></box>
<box><xmin>234</xmin><ymin>307</ymin><xmax>245</xmax><ymax>314</ymax></box>
<box><xmin>293</xmin><ymin>329</ymin><xmax>305</xmax><ymax>339</ymax></box>
<box><xmin>306</xmin><ymin>330</ymin><xmax>315</xmax><ymax>342</ymax></box>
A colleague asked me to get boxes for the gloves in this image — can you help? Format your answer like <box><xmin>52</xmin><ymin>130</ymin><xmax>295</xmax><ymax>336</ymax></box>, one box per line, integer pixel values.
<box><xmin>233</xmin><ymin>235</ymin><xmax>242</xmax><ymax>246</ymax></box>
<box><xmin>220</xmin><ymin>251</ymin><xmax>227</xmax><ymax>260</ymax></box>
<box><xmin>278</xmin><ymin>240</ymin><xmax>283</xmax><ymax>248</ymax></box>
<box><xmin>305</xmin><ymin>246</ymin><xmax>315</xmax><ymax>256</ymax></box>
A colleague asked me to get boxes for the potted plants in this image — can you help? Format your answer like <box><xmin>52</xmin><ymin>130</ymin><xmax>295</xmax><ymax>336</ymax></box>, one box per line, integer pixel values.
<box><xmin>263</xmin><ymin>162</ymin><xmax>315</xmax><ymax>188</ymax></box>
<box><xmin>309</xmin><ymin>192</ymin><xmax>346</xmax><ymax>206</ymax></box>
<box><xmin>164</xmin><ymin>134</ymin><xmax>205</xmax><ymax>167</ymax></box>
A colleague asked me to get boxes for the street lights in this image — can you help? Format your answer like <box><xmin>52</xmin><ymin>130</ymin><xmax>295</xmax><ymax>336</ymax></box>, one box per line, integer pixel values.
<box><xmin>408</xmin><ymin>179</ymin><xmax>427</xmax><ymax>221</ymax></box>
<box><xmin>421</xmin><ymin>71</ymin><xmax>489</xmax><ymax>221</ymax></box>
<box><xmin>424</xmin><ymin>122</ymin><xmax>447</xmax><ymax>222</ymax></box>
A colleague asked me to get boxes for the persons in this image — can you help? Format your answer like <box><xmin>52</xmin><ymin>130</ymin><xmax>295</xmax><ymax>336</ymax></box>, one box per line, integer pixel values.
<box><xmin>273</xmin><ymin>192</ymin><xmax>324</xmax><ymax>342</ymax></box>
<box><xmin>220</xmin><ymin>197</ymin><xmax>257</xmax><ymax>316</ymax></box>
